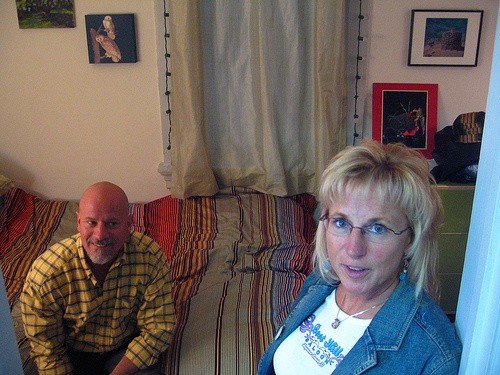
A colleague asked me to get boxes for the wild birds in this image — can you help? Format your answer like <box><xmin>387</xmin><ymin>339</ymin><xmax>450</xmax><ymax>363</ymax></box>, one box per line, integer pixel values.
<box><xmin>95</xmin><ymin>34</ymin><xmax>121</xmax><ymax>63</ymax></box>
<box><xmin>103</xmin><ymin>15</ymin><xmax>116</xmax><ymax>40</ymax></box>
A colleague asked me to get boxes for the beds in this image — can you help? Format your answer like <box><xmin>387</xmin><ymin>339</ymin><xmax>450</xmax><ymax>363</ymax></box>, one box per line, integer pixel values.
<box><xmin>0</xmin><ymin>173</ymin><xmax>318</xmax><ymax>375</ymax></box>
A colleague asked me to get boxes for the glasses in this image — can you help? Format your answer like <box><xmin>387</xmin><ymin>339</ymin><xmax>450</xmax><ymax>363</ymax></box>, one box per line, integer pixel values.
<box><xmin>320</xmin><ymin>213</ymin><xmax>411</xmax><ymax>244</ymax></box>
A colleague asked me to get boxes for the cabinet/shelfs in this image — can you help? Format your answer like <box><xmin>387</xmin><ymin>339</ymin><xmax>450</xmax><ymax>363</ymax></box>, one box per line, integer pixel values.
<box><xmin>431</xmin><ymin>180</ymin><xmax>475</xmax><ymax>314</ymax></box>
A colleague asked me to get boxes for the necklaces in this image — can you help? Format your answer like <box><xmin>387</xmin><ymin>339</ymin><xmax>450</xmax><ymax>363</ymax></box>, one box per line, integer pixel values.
<box><xmin>331</xmin><ymin>293</ymin><xmax>390</xmax><ymax>329</ymax></box>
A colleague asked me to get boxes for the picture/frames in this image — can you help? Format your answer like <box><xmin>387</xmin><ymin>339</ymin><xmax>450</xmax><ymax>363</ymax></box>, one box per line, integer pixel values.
<box><xmin>408</xmin><ymin>9</ymin><xmax>483</xmax><ymax>67</ymax></box>
<box><xmin>373</xmin><ymin>83</ymin><xmax>438</xmax><ymax>159</ymax></box>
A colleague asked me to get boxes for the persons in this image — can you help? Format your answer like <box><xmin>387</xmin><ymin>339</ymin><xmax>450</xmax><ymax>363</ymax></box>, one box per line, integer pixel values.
<box><xmin>258</xmin><ymin>141</ymin><xmax>462</xmax><ymax>375</ymax></box>
<box><xmin>20</xmin><ymin>180</ymin><xmax>177</xmax><ymax>375</ymax></box>
